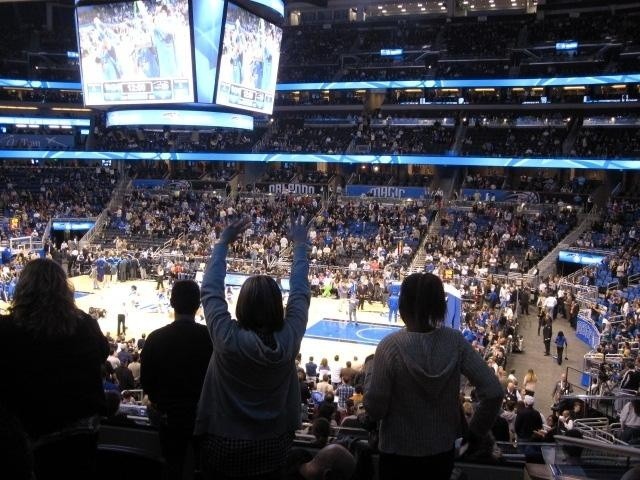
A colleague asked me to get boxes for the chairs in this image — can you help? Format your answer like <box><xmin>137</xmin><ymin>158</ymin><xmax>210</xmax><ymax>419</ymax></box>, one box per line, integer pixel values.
<box><xmin>0</xmin><ymin>379</ymin><xmax>557</xmax><ymax>480</ymax></box>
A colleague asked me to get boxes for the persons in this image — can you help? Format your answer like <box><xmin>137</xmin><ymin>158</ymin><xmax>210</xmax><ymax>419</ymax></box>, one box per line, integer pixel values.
<box><xmin>0</xmin><ymin>0</ymin><xmax>640</xmax><ymax>479</ymax></box>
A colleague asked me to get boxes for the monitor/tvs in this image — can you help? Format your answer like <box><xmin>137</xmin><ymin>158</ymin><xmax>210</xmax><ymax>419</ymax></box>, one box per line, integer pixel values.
<box><xmin>212</xmin><ymin>0</ymin><xmax>283</xmax><ymax>116</ymax></box>
<box><xmin>74</xmin><ymin>0</ymin><xmax>197</xmax><ymax>109</ymax></box>
